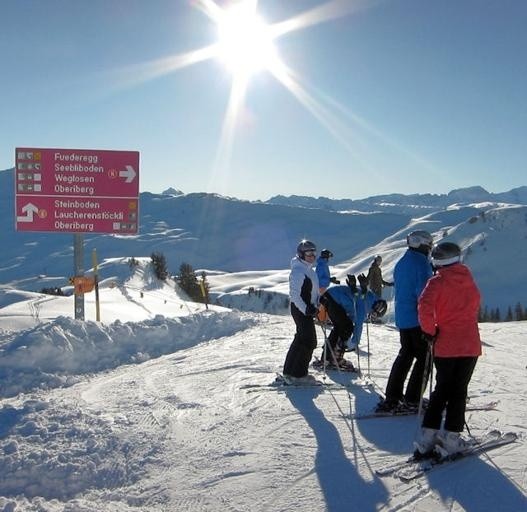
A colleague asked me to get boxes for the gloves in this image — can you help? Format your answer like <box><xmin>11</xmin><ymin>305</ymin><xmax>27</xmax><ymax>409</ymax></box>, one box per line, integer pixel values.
<box><xmin>358</xmin><ymin>273</ymin><xmax>369</xmax><ymax>294</ymax></box>
<box><xmin>345</xmin><ymin>274</ymin><xmax>357</xmax><ymax>293</ymax></box>
<box><xmin>306</xmin><ymin>304</ymin><xmax>319</xmax><ymax>317</ymax></box>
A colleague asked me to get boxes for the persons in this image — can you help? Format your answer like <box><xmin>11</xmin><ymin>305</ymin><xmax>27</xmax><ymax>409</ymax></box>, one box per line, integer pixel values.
<box><xmin>319</xmin><ymin>286</ymin><xmax>388</xmax><ymax>368</ymax></box>
<box><xmin>413</xmin><ymin>242</ymin><xmax>482</xmax><ymax>459</ymax></box>
<box><xmin>367</xmin><ymin>255</ymin><xmax>394</xmax><ymax>298</ymax></box>
<box><xmin>282</xmin><ymin>240</ymin><xmax>320</xmax><ymax>383</ymax></box>
<box><xmin>375</xmin><ymin>230</ymin><xmax>433</xmax><ymax>412</ymax></box>
<box><xmin>316</xmin><ymin>249</ymin><xmax>340</xmax><ymax>322</ymax></box>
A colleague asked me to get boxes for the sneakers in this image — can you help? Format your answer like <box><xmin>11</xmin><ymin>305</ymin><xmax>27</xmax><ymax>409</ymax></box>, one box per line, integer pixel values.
<box><xmin>283</xmin><ymin>375</ymin><xmax>323</xmax><ymax>386</ymax></box>
<box><xmin>321</xmin><ymin>356</ymin><xmax>353</xmax><ymax>369</ymax></box>
<box><xmin>386</xmin><ymin>394</ymin><xmax>429</xmax><ymax>410</ymax></box>
<box><xmin>422</xmin><ymin>428</ymin><xmax>472</xmax><ymax>454</ymax></box>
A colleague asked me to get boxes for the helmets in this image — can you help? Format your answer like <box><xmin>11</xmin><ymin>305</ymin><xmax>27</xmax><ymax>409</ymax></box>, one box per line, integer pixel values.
<box><xmin>432</xmin><ymin>243</ymin><xmax>460</xmax><ymax>269</ymax></box>
<box><xmin>407</xmin><ymin>231</ymin><xmax>431</xmax><ymax>248</ymax></box>
<box><xmin>322</xmin><ymin>249</ymin><xmax>333</xmax><ymax>258</ymax></box>
<box><xmin>297</xmin><ymin>240</ymin><xmax>316</xmax><ymax>258</ymax></box>
<box><xmin>374</xmin><ymin>255</ymin><xmax>382</xmax><ymax>260</ymax></box>
<box><xmin>374</xmin><ymin>300</ymin><xmax>387</xmax><ymax>316</ymax></box>
